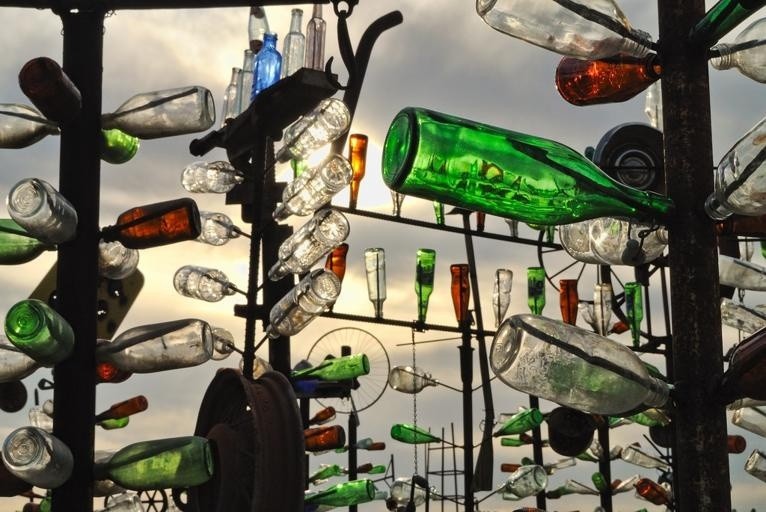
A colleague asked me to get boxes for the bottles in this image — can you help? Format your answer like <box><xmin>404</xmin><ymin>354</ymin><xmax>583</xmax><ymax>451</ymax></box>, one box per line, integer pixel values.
<box><xmin>26</xmin><ymin>393</ymin><xmax>158</xmax><ymax>430</ymax></box>
<box><xmin>479</xmin><ymin>410</ymin><xmax>677</xmax><ymax>510</ymax></box>
<box><xmin>624</xmin><ymin>282</ymin><xmax>645</xmax><ymax>344</ymax></box>
<box><xmin>490</xmin><ymin>267</ymin><xmax>514</xmax><ymax>328</ymax></box>
<box><xmin>0</xmin><ymin>174</ymin><xmax>208</xmax><ymax>279</ymax></box>
<box><xmin>742</xmin><ymin>448</ymin><xmax>764</xmax><ymax>478</ymax></box>
<box><xmin>526</xmin><ymin>265</ymin><xmax>548</xmax><ymax>318</ymax></box>
<box><xmin>449</xmin><ymin>262</ymin><xmax>471</xmax><ymax>324</ymax></box>
<box><xmin>709</xmin><ymin>118</ymin><xmax>764</xmax><ymax>224</ymax></box>
<box><xmin>326</xmin><ymin>244</ymin><xmax>349</xmax><ymax>312</ymax></box>
<box><xmin>176</xmin><ymin>160</ymin><xmax>233</xmax><ymax>363</ymax></box>
<box><xmin>505</xmin><ymin>217</ymin><xmax>520</xmax><ymax>239</ymax></box>
<box><xmin>732</xmin><ymin>402</ymin><xmax>765</xmax><ymax>437</ymax></box>
<box><xmin>371</xmin><ymin>107</ymin><xmax>676</xmax><ymax>234</ymax></box>
<box><xmin>726</xmin><ymin>434</ymin><xmax>743</xmax><ymax>459</ymax></box>
<box><xmin>385</xmin><ymin>191</ymin><xmax>406</xmax><ymax>219</ymax></box>
<box><xmin>475</xmin><ymin>0</ymin><xmax>662</xmax><ymax>60</ymax></box>
<box><xmin>553</xmin><ymin>51</ymin><xmax>672</xmax><ymax>108</ymax></box>
<box><xmin>262</xmin><ymin>96</ymin><xmax>349</xmax><ymax>350</ymax></box>
<box><xmin>708</xmin><ymin>21</ymin><xmax>766</xmax><ymax>90</ymax></box>
<box><xmin>592</xmin><ymin>281</ymin><xmax>613</xmax><ymax>337</ymax></box>
<box><xmin>291</xmin><ymin>352</ymin><xmax>389</xmax><ymax>512</ymax></box>
<box><xmin>344</xmin><ymin>132</ymin><xmax>366</xmax><ymax>212</ymax></box>
<box><xmin>220</xmin><ymin>0</ymin><xmax>326</xmax><ymax>124</ymax></box>
<box><xmin>429</xmin><ymin>196</ymin><xmax>444</xmax><ymax>225</ymax></box>
<box><xmin>715</xmin><ymin>328</ymin><xmax>766</xmax><ymax>409</ymax></box>
<box><xmin>1</xmin><ymin>58</ymin><xmax>213</xmax><ymax>167</ymax></box>
<box><xmin>414</xmin><ymin>248</ymin><xmax>437</xmax><ymax>322</ymax></box>
<box><xmin>476</xmin><ymin>212</ymin><xmax>487</xmax><ymax>232</ymax></box>
<box><xmin>1</xmin><ymin>296</ymin><xmax>218</xmax><ymax>391</ymax></box>
<box><xmin>389</xmin><ymin>367</ymin><xmax>444</xmax><ymax>505</ymax></box>
<box><xmin>482</xmin><ymin>309</ymin><xmax>672</xmax><ymax>413</ymax></box>
<box><xmin>545</xmin><ymin>223</ymin><xmax>556</xmax><ymax>243</ymax></box>
<box><xmin>0</xmin><ymin>427</ymin><xmax>219</xmax><ymax>501</ymax></box>
<box><xmin>558</xmin><ymin>278</ymin><xmax>581</xmax><ymax>325</ymax></box>
<box><xmin>363</xmin><ymin>248</ymin><xmax>388</xmax><ymax>318</ymax></box>
